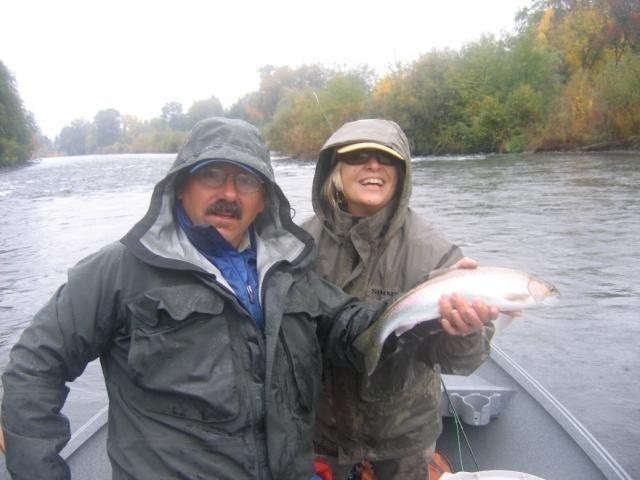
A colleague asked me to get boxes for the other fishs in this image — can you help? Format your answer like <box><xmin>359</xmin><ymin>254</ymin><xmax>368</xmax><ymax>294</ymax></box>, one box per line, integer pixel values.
<box><xmin>352</xmin><ymin>266</ymin><xmax>563</xmax><ymax>376</ymax></box>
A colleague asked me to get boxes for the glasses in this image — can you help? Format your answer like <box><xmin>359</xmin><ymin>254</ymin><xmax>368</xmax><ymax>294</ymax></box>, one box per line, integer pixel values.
<box><xmin>339</xmin><ymin>150</ymin><xmax>398</xmax><ymax>165</ymax></box>
<box><xmin>196</xmin><ymin>166</ymin><xmax>264</xmax><ymax>195</ymax></box>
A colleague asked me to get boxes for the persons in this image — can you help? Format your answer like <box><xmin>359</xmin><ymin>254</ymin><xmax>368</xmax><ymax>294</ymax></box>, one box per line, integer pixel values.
<box><xmin>305</xmin><ymin>118</ymin><xmax>500</xmax><ymax>480</ymax></box>
<box><xmin>0</xmin><ymin>117</ymin><xmax>523</xmax><ymax>480</ymax></box>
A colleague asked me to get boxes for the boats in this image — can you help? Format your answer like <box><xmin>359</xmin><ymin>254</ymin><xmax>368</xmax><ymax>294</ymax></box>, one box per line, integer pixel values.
<box><xmin>57</xmin><ymin>342</ymin><xmax>632</xmax><ymax>480</ymax></box>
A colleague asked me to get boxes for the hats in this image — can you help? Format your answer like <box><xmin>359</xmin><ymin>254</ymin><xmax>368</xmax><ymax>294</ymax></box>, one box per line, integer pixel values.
<box><xmin>337</xmin><ymin>142</ymin><xmax>406</xmax><ymax>160</ymax></box>
<box><xmin>190</xmin><ymin>160</ymin><xmax>261</xmax><ymax>178</ymax></box>
